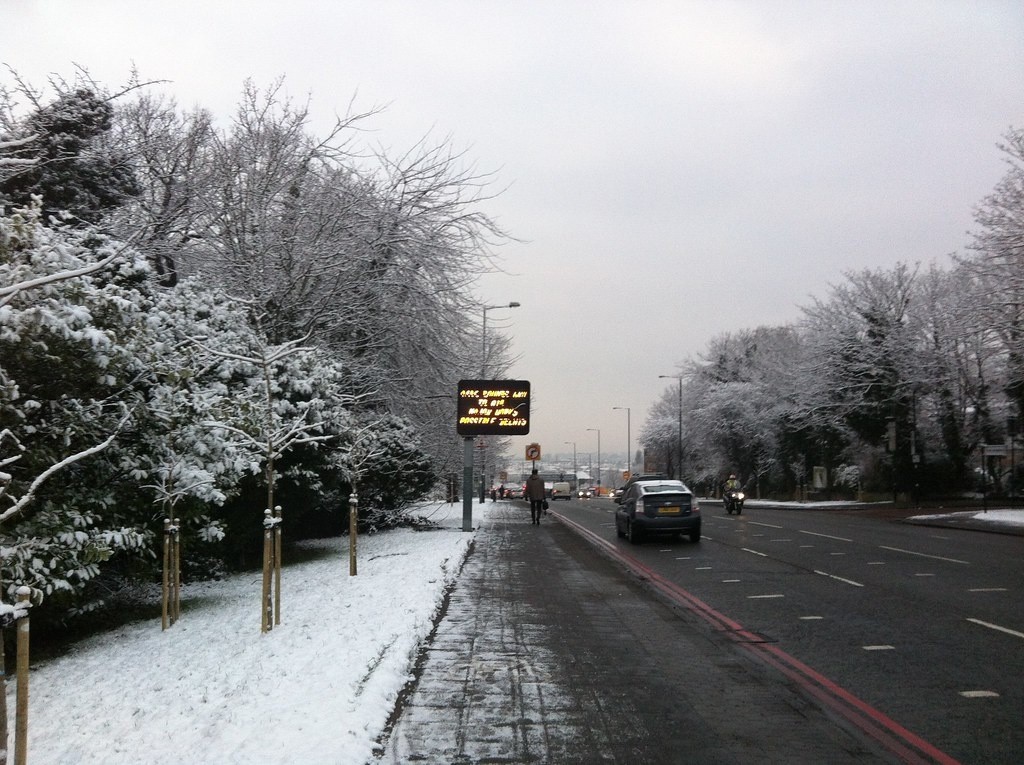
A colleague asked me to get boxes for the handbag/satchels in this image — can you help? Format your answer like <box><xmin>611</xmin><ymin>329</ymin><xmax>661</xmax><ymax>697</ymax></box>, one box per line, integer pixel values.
<box><xmin>543</xmin><ymin>499</ymin><xmax>548</xmax><ymax>510</ymax></box>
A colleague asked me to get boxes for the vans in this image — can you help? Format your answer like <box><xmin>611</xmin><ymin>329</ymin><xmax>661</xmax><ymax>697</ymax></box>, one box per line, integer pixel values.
<box><xmin>551</xmin><ymin>483</ymin><xmax>571</xmax><ymax>500</ymax></box>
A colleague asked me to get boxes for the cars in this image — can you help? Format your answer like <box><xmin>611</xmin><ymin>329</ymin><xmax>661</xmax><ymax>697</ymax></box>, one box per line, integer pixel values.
<box><xmin>510</xmin><ymin>487</ymin><xmax>524</xmax><ymax>499</ymax></box>
<box><xmin>544</xmin><ymin>483</ymin><xmax>553</xmax><ymax>496</ymax></box>
<box><xmin>613</xmin><ymin>478</ymin><xmax>702</xmax><ymax>543</ymax></box>
<box><xmin>489</xmin><ymin>487</ymin><xmax>507</xmax><ymax>498</ymax></box>
<box><xmin>577</xmin><ymin>489</ymin><xmax>591</xmax><ymax>498</ymax></box>
<box><xmin>504</xmin><ymin>483</ymin><xmax>520</xmax><ymax>496</ymax></box>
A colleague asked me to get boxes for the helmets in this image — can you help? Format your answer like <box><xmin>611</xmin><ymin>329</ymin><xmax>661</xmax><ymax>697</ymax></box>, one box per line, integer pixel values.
<box><xmin>730</xmin><ymin>475</ymin><xmax>736</xmax><ymax>478</ymax></box>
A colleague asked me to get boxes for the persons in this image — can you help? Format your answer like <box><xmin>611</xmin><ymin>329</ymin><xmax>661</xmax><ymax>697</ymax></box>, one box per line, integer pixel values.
<box><xmin>722</xmin><ymin>475</ymin><xmax>742</xmax><ymax>509</ymax></box>
<box><xmin>526</xmin><ymin>469</ymin><xmax>546</xmax><ymax>525</ymax></box>
<box><xmin>498</xmin><ymin>485</ymin><xmax>505</xmax><ymax>500</ymax></box>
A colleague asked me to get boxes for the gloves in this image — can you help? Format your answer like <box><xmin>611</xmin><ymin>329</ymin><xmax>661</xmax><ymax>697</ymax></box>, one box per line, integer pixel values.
<box><xmin>525</xmin><ymin>495</ymin><xmax>529</xmax><ymax>501</ymax></box>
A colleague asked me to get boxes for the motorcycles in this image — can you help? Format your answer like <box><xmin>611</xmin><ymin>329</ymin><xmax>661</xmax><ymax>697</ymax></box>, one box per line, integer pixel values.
<box><xmin>723</xmin><ymin>484</ymin><xmax>748</xmax><ymax>515</ymax></box>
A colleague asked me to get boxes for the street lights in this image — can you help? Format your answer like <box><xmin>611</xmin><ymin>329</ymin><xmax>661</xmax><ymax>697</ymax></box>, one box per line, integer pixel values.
<box><xmin>481</xmin><ymin>302</ymin><xmax>521</xmax><ymax>501</ymax></box>
<box><xmin>613</xmin><ymin>407</ymin><xmax>631</xmax><ymax>480</ymax></box>
<box><xmin>586</xmin><ymin>429</ymin><xmax>600</xmax><ymax>496</ymax></box>
<box><xmin>658</xmin><ymin>375</ymin><xmax>683</xmax><ymax>481</ymax></box>
<box><xmin>564</xmin><ymin>442</ymin><xmax>576</xmax><ymax>492</ymax></box>
